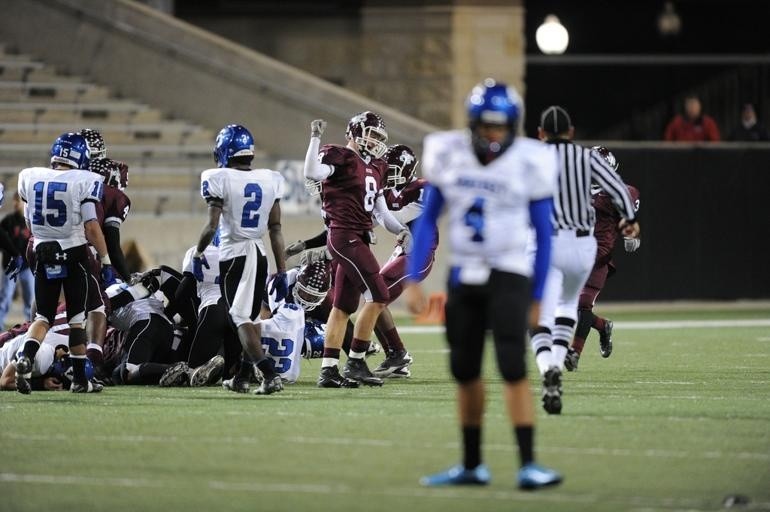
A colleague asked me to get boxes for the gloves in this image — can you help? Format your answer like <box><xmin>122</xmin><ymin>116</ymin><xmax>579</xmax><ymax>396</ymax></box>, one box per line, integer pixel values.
<box><xmin>311</xmin><ymin>119</ymin><xmax>327</xmax><ymax>138</ymax></box>
<box><xmin>624</xmin><ymin>235</ymin><xmax>641</xmax><ymax>252</ymax></box>
<box><xmin>192</xmin><ymin>254</ymin><xmax>210</xmax><ymax>282</ymax></box>
<box><xmin>101</xmin><ymin>255</ymin><xmax>115</xmax><ymax>283</ymax></box>
<box><xmin>270</xmin><ymin>274</ymin><xmax>289</xmax><ymax>301</ymax></box>
<box><xmin>284</xmin><ymin>240</ymin><xmax>305</xmax><ymax>261</ymax></box>
<box><xmin>395</xmin><ymin>229</ymin><xmax>415</xmax><ymax>254</ymax></box>
<box><xmin>4</xmin><ymin>256</ymin><xmax>24</xmax><ymax>283</ymax></box>
<box><xmin>301</xmin><ymin>249</ymin><xmax>332</xmax><ymax>263</ymax></box>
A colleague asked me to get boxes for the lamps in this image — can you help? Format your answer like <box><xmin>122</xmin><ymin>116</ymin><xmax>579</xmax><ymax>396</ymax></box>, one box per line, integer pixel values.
<box><xmin>535</xmin><ymin>14</ymin><xmax>569</xmax><ymax>54</ymax></box>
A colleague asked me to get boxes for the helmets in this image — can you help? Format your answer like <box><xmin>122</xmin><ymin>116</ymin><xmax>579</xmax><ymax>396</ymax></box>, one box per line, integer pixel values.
<box><xmin>589</xmin><ymin>146</ymin><xmax>618</xmax><ymax>187</ymax></box>
<box><xmin>293</xmin><ymin>260</ymin><xmax>329</xmax><ymax>313</ymax></box>
<box><xmin>345</xmin><ymin>110</ymin><xmax>388</xmax><ymax>161</ymax></box>
<box><xmin>464</xmin><ymin>81</ymin><xmax>523</xmax><ymax>164</ymax></box>
<box><xmin>384</xmin><ymin>143</ymin><xmax>418</xmax><ymax>189</ymax></box>
<box><xmin>213</xmin><ymin>124</ymin><xmax>255</xmax><ymax>167</ymax></box>
<box><xmin>303</xmin><ymin>321</ymin><xmax>328</xmax><ymax>360</ymax></box>
<box><xmin>55</xmin><ymin>355</ymin><xmax>93</xmax><ymax>386</ymax></box>
<box><xmin>49</xmin><ymin>127</ymin><xmax>122</xmax><ymax>188</ymax></box>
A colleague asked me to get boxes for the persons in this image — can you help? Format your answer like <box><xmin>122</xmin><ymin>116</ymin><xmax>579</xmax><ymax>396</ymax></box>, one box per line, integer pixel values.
<box><xmin>1</xmin><ymin>110</ymin><xmax>439</xmax><ymax>395</ymax></box>
<box><xmin>532</xmin><ymin>108</ymin><xmax>638</xmax><ymax>414</ymax></box>
<box><xmin>720</xmin><ymin>98</ymin><xmax>770</xmax><ymax>142</ymax></box>
<box><xmin>562</xmin><ymin>145</ymin><xmax>639</xmax><ymax>372</ymax></box>
<box><xmin>661</xmin><ymin>94</ymin><xmax>722</xmax><ymax>141</ymax></box>
<box><xmin>400</xmin><ymin>79</ymin><xmax>563</xmax><ymax>490</ymax></box>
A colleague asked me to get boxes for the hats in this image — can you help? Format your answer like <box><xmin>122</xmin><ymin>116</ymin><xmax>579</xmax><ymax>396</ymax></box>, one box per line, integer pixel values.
<box><xmin>541</xmin><ymin>106</ymin><xmax>570</xmax><ymax>135</ymax></box>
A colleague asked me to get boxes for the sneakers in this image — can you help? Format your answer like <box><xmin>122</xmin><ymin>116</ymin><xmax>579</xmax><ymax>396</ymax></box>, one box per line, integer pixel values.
<box><xmin>365</xmin><ymin>341</ymin><xmax>382</xmax><ymax>356</ymax></box>
<box><xmin>565</xmin><ymin>346</ymin><xmax>579</xmax><ymax>371</ymax></box>
<box><xmin>14</xmin><ymin>355</ymin><xmax>33</xmax><ymax>394</ymax></box>
<box><xmin>70</xmin><ymin>382</ymin><xmax>103</xmax><ymax>393</ymax></box>
<box><xmin>318</xmin><ymin>364</ymin><xmax>359</xmax><ymax>388</ymax></box>
<box><xmin>342</xmin><ymin>357</ymin><xmax>384</xmax><ymax>386</ymax></box>
<box><xmin>190</xmin><ymin>355</ymin><xmax>225</xmax><ymax>387</ymax></box>
<box><xmin>373</xmin><ymin>353</ymin><xmax>416</xmax><ymax>378</ymax></box>
<box><xmin>422</xmin><ymin>464</ymin><xmax>490</xmax><ymax>485</ymax></box>
<box><xmin>254</xmin><ymin>374</ymin><xmax>283</xmax><ymax>395</ymax></box>
<box><xmin>222</xmin><ymin>378</ymin><xmax>250</xmax><ymax>393</ymax></box>
<box><xmin>597</xmin><ymin>318</ymin><xmax>612</xmax><ymax>357</ymax></box>
<box><xmin>518</xmin><ymin>463</ymin><xmax>562</xmax><ymax>489</ymax></box>
<box><xmin>542</xmin><ymin>366</ymin><xmax>563</xmax><ymax>414</ymax></box>
<box><xmin>158</xmin><ymin>361</ymin><xmax>188</xmax><ymax>387</ymax></box>
<box><xmin>85</xmin><ymin>348</ymin><xmax>108</xmax><ymax>379</ymax></box>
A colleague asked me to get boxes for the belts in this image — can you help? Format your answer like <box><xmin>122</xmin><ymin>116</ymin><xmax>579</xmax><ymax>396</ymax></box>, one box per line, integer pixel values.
<box><xmin>552</xmin><ymin>228</ymin><xmax>588</xmax><ymax>237</ymax></box>
